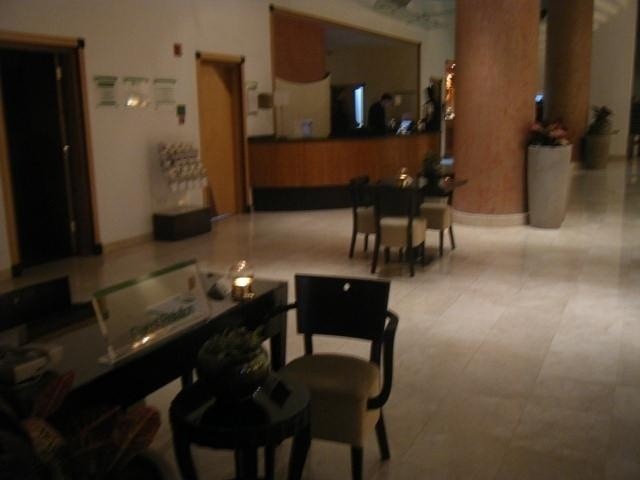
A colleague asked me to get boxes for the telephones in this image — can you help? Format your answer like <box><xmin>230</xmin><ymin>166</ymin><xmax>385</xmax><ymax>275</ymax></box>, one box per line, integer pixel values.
<box><xmin>0</xmin><ymin>342</ymin><xmax>63</xmax><ymax>386</ymax></box>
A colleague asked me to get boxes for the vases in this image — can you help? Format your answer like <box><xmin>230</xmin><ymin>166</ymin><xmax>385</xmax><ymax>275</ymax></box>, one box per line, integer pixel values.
<box><xmin>527</xmin><ymin>144</ymin><xmax>573</xmax><ymax>228</ymax></box>
<box><xmin>584</xmin><ymin>134</ymin><xmax>610</xmax><ymax>168</ymax></box>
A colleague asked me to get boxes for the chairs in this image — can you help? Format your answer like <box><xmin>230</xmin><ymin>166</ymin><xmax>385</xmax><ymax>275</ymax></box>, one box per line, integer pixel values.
<box><xmin>345</xmin><ymin>172</ymin><xmax>456</xmax><ymax>277</ymax></box>
<box><xmin>247</xmin><ymin>272</ymin><xmax>400</xmax><ymax>479</ymax></box>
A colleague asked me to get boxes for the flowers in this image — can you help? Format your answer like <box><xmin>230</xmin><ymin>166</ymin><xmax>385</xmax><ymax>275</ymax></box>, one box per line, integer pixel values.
<box><xmin>532</xmin><ymin>121</ymin><xmax>569</xmax><ymax>147</ymax></box>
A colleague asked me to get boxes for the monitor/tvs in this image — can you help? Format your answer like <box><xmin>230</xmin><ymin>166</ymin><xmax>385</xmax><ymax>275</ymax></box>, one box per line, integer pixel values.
<box><xmin>534</xmin><ymin>94</ymin><xmax>544</xmax><ymax>102</ymax></box>
<box><xmin>394</xmin><ymin>120</ymin><xmax>416</xmax><ymax>135</ymax></box>
<box><xmin>351</xmin><ymin>83</ymin><xmax>365</xmax><ymax>128</ymax></box>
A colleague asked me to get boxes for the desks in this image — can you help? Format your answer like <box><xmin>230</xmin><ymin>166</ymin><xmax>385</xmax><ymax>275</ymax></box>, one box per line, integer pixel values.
<box><xmin>1</xmin><ymin>273</ymin><xmax>287</xmax><ymax>480</ymax></box>
<box><xmin>169</xmin><ymin>381</ymin><xmax>268</xmax><ymax>480</ymax></box>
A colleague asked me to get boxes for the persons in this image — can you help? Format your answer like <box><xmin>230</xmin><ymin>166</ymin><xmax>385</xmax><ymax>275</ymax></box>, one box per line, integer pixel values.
<box><xmin>367</xmin><ymin>91</ymin><xmax>397</xmax><ymax>135</ymax></box>
<box><xmin>417</xmin><ymin>85</ymin><xmax>441</xmax><ymax>133</ymax></box>
